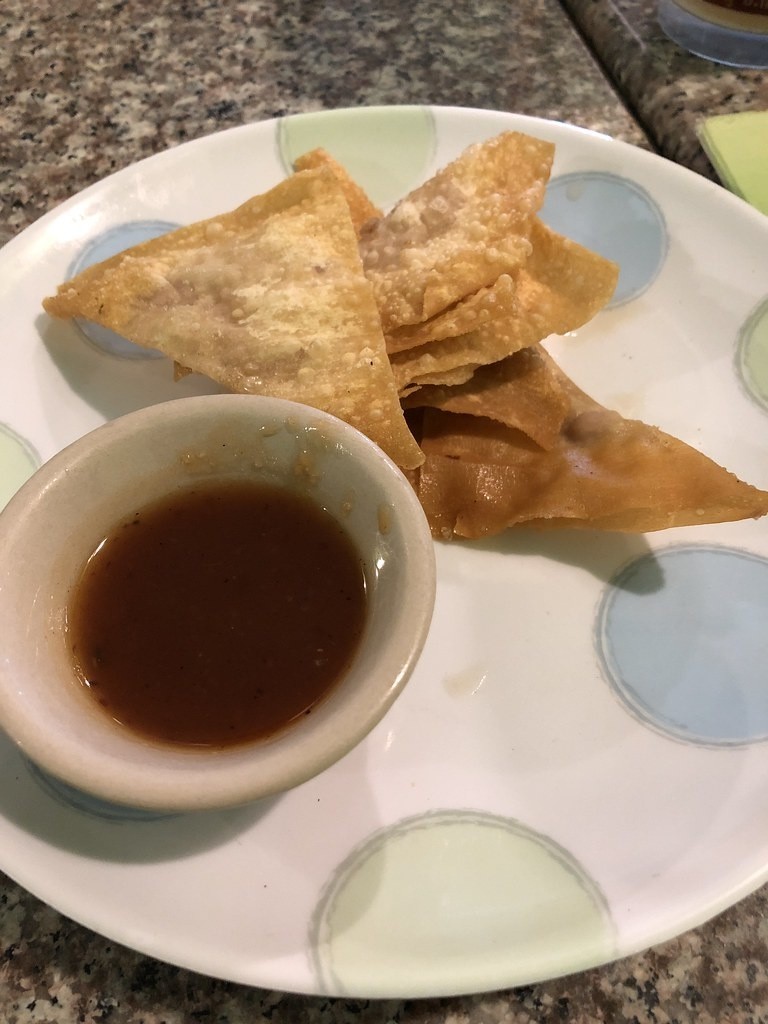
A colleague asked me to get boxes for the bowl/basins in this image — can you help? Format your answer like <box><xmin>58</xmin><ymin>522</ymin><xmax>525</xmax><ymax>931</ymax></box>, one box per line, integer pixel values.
<box><xmin>0</xmin><ymin>392</ymin><xmax>437</xmax><ymax>813</ymax></box>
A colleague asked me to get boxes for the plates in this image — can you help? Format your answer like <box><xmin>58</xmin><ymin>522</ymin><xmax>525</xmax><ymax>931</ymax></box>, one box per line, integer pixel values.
<box><xmin>0</xmin><ymin>104</ymin><xmax>767</xmax><ymax>1000</ymax></box>
